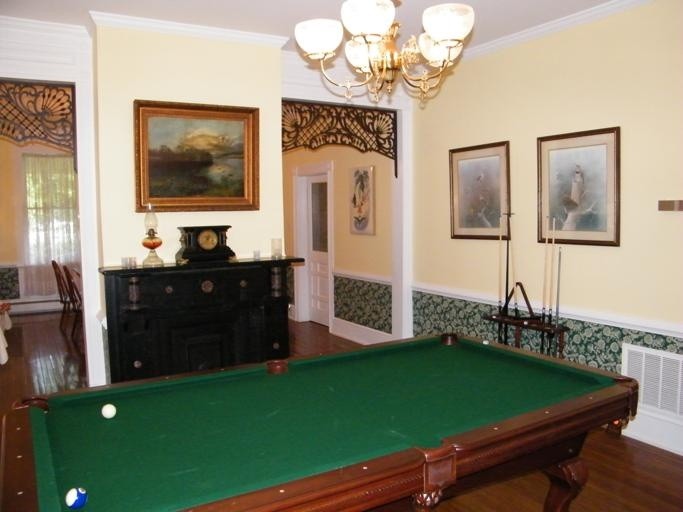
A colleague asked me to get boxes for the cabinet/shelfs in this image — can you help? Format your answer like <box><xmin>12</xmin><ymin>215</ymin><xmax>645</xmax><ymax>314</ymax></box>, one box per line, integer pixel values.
<box><xmin>98</xmin><ymin>265</ymin><xmax>288</xmax><ymax>385</ymax></box>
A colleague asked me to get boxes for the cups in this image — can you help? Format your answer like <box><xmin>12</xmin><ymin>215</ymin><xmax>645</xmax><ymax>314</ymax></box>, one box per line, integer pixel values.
<box><xmin>270</xmin><ymin>237</ymin><xmax>283</xmax><ymax>259</ymax></box>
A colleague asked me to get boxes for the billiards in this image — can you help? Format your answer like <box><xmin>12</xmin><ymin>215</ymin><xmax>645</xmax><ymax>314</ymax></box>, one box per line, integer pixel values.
<box><xmin>100</xmin><ymin>404</ymin><xmax>116</xmax><ymax>419</ymax></box>
<box><xmin>65</xmin><ymin>487</ymin><xmax>88</xmax><ymax>509</ymax></box>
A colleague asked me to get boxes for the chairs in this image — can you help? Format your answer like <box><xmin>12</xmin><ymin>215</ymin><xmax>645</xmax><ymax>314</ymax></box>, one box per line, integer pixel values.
<box><xmin>50</xmin><ymin>261</ymin><xmax>82</xmax><ymax>340</ymax></box>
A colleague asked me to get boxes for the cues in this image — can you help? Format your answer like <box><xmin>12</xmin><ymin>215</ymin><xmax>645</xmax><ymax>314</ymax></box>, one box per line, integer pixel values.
<box><xmin>541</xmin><ymin>216</ymin><xmax>562</xmax><ymax>357</ymax></box>
<box><xmin>499</xmin><ymin>213</ymin><xmax>520</xmax><ymax>349</ymax></box>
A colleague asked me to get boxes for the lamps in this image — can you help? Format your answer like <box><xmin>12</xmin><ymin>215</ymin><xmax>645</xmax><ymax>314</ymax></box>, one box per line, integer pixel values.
<box><xmin>294</xmin><ymin>1</ymin><xmax>476</xmax><ymax>105</ymax></box>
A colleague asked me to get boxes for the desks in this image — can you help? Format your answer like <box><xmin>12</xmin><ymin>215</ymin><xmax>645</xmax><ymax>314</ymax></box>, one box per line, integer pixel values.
<box><xmin>0</xmin><ymin>333</ymin><xmax>640</xmax><ymax>512</ymax></box>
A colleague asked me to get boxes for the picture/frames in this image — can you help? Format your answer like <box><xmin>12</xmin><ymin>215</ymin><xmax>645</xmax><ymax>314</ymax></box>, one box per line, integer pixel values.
<box><xmin>448</xmin><ymin>127</ymin><xmax>620</xmax><ymax>247</ymax></box>
<box><xmin>133</xmin><ymin>101</ymin><xmax>260</xmax><ymax>213</ymax></box>
